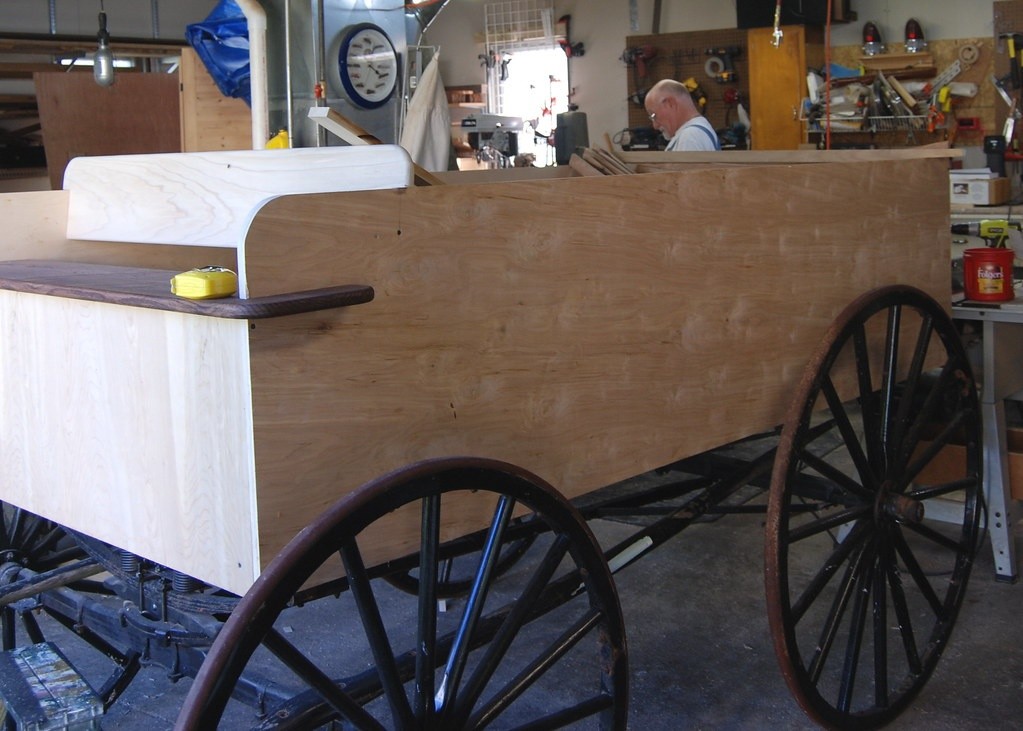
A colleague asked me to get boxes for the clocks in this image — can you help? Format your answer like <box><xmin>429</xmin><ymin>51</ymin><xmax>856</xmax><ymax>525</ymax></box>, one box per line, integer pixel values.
<box><xmin>327</xmin><ymin>20</ymin><xmax>400</xmax><ymax>109</ymax></box>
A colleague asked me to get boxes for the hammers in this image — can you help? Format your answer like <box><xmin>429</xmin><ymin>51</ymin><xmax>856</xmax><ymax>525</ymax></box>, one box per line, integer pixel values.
<box><xmin>999</xmin><ymin>32</ymin><xmax>1023</xmax><ymax>89</ymax></box>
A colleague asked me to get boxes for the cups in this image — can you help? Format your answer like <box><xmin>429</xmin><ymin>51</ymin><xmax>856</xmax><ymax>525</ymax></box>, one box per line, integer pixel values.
<box><xmin>963</xmin><ymin>247</ymin><xmax>1016</xmax><ymax>301</ymax></box>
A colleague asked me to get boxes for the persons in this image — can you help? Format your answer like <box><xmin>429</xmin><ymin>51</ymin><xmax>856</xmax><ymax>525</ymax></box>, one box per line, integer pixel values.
<box><xmin>644</xmin><ymin>79</ymin><xmax>721</xmax><ymax>151</ymax></box>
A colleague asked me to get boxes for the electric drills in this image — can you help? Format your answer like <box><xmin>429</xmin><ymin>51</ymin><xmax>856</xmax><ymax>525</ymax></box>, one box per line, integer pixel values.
<box><xmin>951</xmin><ymin>219</ymin><xmax>1023</xmax><ymax>248</ymax></box>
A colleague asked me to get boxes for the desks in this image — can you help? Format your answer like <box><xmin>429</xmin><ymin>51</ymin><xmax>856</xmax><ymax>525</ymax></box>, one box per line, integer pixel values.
<box><xmin>834</xmin><ymin>279</ymin><xmax>1023</xmax><ymax>581</ymax></box>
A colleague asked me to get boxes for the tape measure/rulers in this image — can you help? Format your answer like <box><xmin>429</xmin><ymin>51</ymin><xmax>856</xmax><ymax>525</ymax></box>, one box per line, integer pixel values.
<box><xmin>169</xmin><ymin>265</ymin><xmax>238</xmax><ymax>299</ymax></box>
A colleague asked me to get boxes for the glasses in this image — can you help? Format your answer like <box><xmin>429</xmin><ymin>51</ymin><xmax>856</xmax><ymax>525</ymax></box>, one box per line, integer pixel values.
<box><xmin>647</xmin><ymin>98</ymin><xmax>669</xmax><ymax>121</ymax></box>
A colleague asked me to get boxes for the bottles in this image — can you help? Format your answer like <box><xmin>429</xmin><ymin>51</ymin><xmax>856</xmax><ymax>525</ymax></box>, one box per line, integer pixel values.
<box><xmin>264</xmin><ymin>129</ymin><xmax>289</xmax><ymax>149</ymax></box>
